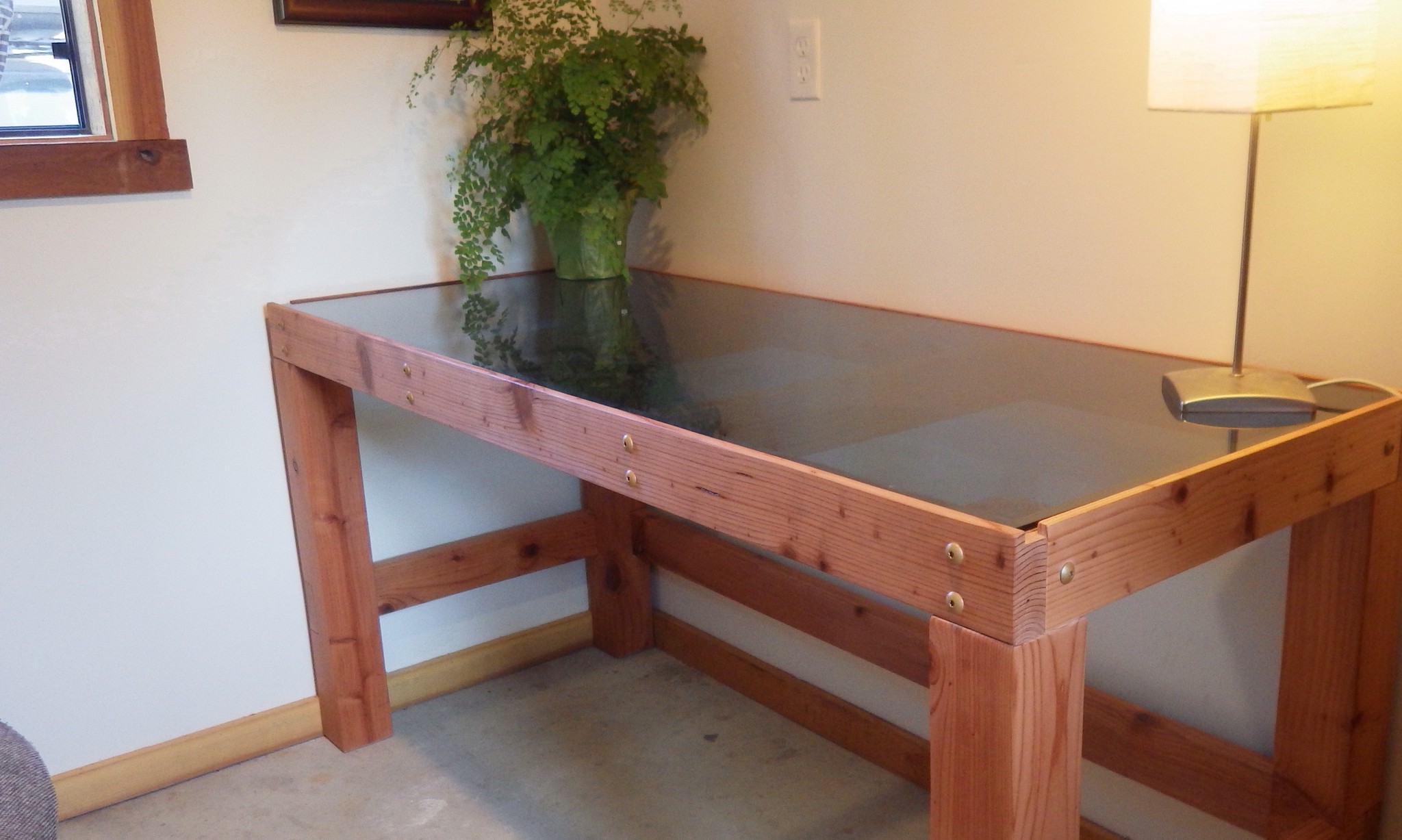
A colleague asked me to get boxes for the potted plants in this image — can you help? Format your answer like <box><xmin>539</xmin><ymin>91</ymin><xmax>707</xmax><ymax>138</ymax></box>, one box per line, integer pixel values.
<box><xmin>406</xmin><ymin>0</ymin><xmax>711</xmax><ymax>295</ymax></box>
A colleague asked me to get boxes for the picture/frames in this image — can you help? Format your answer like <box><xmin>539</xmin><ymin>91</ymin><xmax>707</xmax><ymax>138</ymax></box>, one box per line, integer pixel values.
<box><xmin>272</xmin><ymin>0</ymin><xmax>494</xmax><ymax>32</ymax></box>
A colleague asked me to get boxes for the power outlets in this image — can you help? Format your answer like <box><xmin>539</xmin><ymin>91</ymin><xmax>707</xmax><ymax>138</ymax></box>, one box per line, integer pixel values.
<box><xmin>790</xmin><ymin>19</ymin><xmax>822</xmax><ymax>101</ymax></box>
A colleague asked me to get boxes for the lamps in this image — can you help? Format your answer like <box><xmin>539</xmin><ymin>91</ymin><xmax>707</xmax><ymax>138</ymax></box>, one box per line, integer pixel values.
<box><xmin>1147</xmin><ymin>0</ymin><xmax>1374</xmax><ymax>413</ymax></box>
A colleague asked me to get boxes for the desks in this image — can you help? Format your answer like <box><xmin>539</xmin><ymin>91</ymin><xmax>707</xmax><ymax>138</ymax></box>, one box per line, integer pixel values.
<box><xmin>263</xmin><ymin>268</ymin><xmax>1401</xmax><ymax>840</ymax></box>
